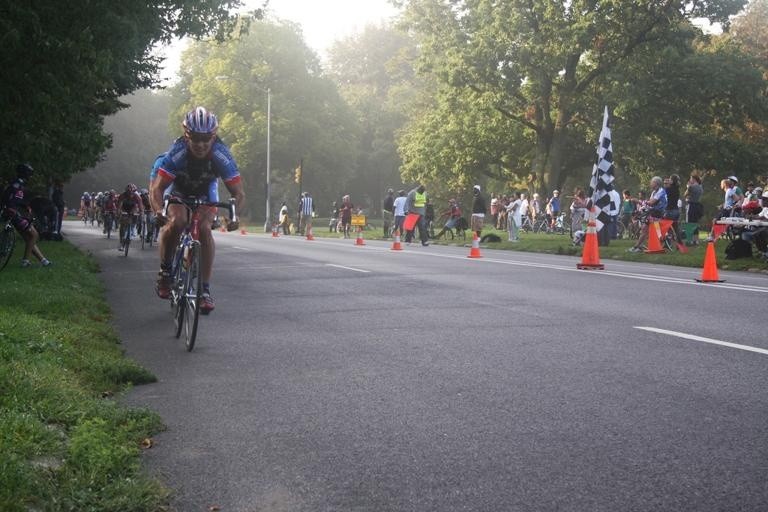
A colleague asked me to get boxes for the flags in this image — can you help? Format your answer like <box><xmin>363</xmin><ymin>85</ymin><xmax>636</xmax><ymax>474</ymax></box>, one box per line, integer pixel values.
<box><xmin>588</xmin><ymin>104</ymin><xmax>615</xmax><ymax>246</ymax></box>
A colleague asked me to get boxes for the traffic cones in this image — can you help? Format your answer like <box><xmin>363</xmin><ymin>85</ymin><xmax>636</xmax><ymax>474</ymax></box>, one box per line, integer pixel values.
<box><xmin>305</xmin><ymin>223</ymin><xmax>316</xmax><ymax>241</ymax></box>
<box><xmin>353</xmin><ymin>227</ymin><xmax>367</xmax><ymax>246</ymax></box>
<box><xmin>466</xmin><ymin>232</ymin><xmax>482</xmax><ymax>259</ymax></box>
<box><xmin>388</xmin><ymin>229</ymin><xmax>404</xmax><ymax>251</ymax></box>
<box><xmin>641</xmin><ymin>219</ymin><xmax>664</xmax><ymax>254</ymax></box>
<box><xmin>576</xmin><ymin>207</ymin><xmax>605</xmax><ymax>269</ymax></box>
<box><xmin>271</xmin><ymin>221</ymin><xmax>281</xmax><ymax>238</ymax></box>
<box><xmin>692</xmin><ymin>239</ymin><xmax>727</xmax><ymax>283</ymax></box>
<box><xmin>240</xmin><ymin>222</ymin><xmax>246</xmax><ymax>235</ymax></box>
<box><xmin>219</xmin><ymin>223</ymin><xmax>226</xmax><ymax>233</ymax></box>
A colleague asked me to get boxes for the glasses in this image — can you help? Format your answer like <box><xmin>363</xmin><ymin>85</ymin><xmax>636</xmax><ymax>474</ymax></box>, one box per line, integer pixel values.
<box><xmin>187</xmin><ymin>132</ymin><xmax>213</xmax><ymax>143</ymax></box>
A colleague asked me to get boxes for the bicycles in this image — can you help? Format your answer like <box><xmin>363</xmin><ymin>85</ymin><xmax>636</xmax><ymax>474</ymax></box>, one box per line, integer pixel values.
<box><xmin>518</xmin><ymin>213</ymin><xmax>569</xmax><ymax>235</ymax></box>
<box><xmin>158</xmin><ymin>194</ymin><xmax>238</xmax><ymax>352</ymax></box>
<box><xmin>424</xmin><ymin>216</ymin><xmax>467</xmax><ymax>243</ymax></box>
<box><xmin>636</xmin><ymin>212</ymin><xmax>678</xmax><ymax>252</ymax></box>
<box><xmin>0</xmin><ymin>209</ymin><xmax>38</xmax><ymax>272</ymax></box>
<box><xmin>615</xmin><ymin>215</ymin><xmax>638</xmax><ymax>242</ymax></box>
<box><xmin>79</xmin><ymin>206</ymin><xmax>157</xmax><ymax>258</ymax></box>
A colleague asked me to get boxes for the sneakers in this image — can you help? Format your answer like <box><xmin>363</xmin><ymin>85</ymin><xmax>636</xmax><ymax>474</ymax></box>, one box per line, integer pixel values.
<box><xmin>21</xmin><ymin>260</ymin><xmax>33</xmax><ymax>267</ymax></box>
<box><xmin>40</xmin><ymin>257</ymin><xmax>50</xmax><ymax>266</ymax></box>
<box><xmin>155</xmin><ymin>270</ymin><xmax>172</xmax><ymax>298</ymax></box>
<box><xmin>199</xmin><ymin>289</ymin><xmax>215</xmax><ymax>311</ymax></box>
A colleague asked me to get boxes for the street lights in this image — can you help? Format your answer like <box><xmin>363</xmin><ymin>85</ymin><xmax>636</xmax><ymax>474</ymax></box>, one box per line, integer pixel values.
<box><xmin>293</xmin><ymin>158</ymin><xmax>304</xmax><ymax>234</ymax></box>
<box><xmin>214</xmin><ymin>74</ymin><xmax>274</xmax><ymax>234</ymax></box>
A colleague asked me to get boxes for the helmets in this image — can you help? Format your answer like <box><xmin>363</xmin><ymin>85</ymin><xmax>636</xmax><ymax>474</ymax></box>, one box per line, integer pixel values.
<box><xmin>183</xmin><ymin>107</ymin><xmax>218</xmax><ymax>135</ymax></box>
<box><xmin>17</xmin><ymin>165</ymin><xmax>34</xmax><ymax>184</ymax></box>
<box><xmin>728</xmin><ymin>176</ymin><xmax>738</xmax><ymax>183</ymax></box>
<box><xmin>125</xmin><ymin>182</ymin><xmax>138</xmax><ymax>193</ymax></box>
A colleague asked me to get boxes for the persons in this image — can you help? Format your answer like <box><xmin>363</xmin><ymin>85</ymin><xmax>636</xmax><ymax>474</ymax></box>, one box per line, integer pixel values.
<box><xmin>150</xmin><ymin>105</ymin><xmax>245</xmax><ymax>313</ymax></box>
<box><xmin>277</xmin><ymin>192</ymin><xmax>315</xmax><ymax>236</ymax></box>
<box><xmin>615</xmin><ymin>174</ymin><xmax>703</xmax><ymax>254</ymax></box>
<box><xmin>383</xmin><ymin>186</ymin><xmax>429</xmax><ymax>246</ymax></box>
<box><xmin>706</xmin><ymin>176</ymin><xmax>767</xmax><ymax>243</ymax></box>
<box><xmin>80</xmin><ymin>183</ymin><xmax>161</xmax><ymax>252</ymax></box>
<box><xmin>432</xmin><ymin>185</ymin><xmax>487</xmax><ymax>244</ymax></box>
<box><xmin>491</xmin><ymin>190</ymin><xmax>565</xmax><ymax>242</ymax></box>
<box><xmin>330</xmin><ymin>195</ymin><xmax>363</xmax><ymax>239</ymax></box>
<box><xmin>0</xmin><ymin>163</ymin><xmax>52</xmax><ymax>268</ymax></box>
<box><xmin>567</xmin><ymin>187</ymin><xmax>585</xmax><ymax>242</ymax></box>
